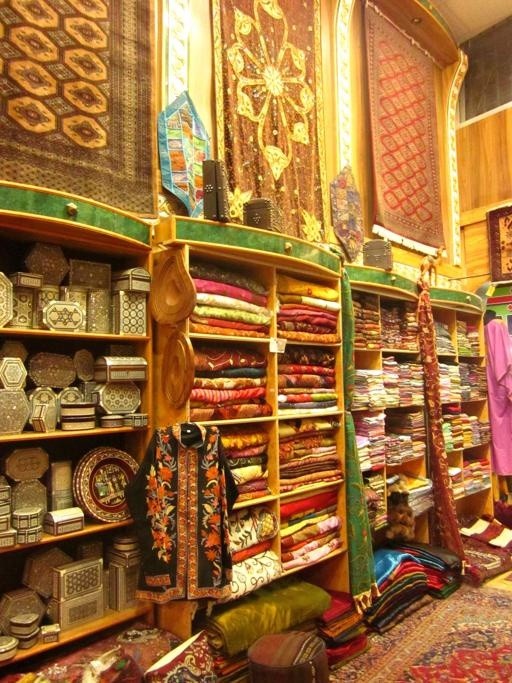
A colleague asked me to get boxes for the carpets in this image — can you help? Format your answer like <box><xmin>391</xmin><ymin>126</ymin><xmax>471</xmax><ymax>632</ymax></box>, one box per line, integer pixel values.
<box><xmin>209</xmin><ymin>0</ymin><xmax>341</xmax><ymax>246</ymax></box>
<box><xmin>361</xmin><ymin>0</ymin><xmax>447</xmax><ymax>257</ymax></box>
<box><xmin>328</xmin><ymin>584</ymin><xmax>512</xmax><ymax>683</ymax></box>
<box><xmin>0</xmin><ymin>1</ymin><xmax>165</xmax><ymax>220</ymax></box>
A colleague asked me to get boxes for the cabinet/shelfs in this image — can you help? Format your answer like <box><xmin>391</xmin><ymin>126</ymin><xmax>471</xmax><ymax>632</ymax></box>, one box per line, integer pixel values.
<box><xmin>0</xmin><ymin>178</ymin><xmax>155</xmax><ymax>671</ymax></box>
<box><xmin>157</xmin><ymin>214</ymin><xmax>350</xmax><ymax>613</ymax></box>
<box><xmin>344</xmin><ymin>261</ymin><xmax>431</xmax><ymax>549</ymax></box>
<box><xmin>427</xmin><ymin>284</ymin><xmax>496</xmax><ymax>519</ymax></box>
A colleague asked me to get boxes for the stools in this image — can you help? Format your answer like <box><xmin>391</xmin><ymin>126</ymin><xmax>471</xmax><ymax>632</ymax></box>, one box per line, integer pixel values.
<box><xmin>245</xmin><ymin>629</ymin><xmax>331</xmax><ymax>683</ymax></box>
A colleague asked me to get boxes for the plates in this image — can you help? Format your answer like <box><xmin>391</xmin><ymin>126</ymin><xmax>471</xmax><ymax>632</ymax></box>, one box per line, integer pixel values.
<box><xmin>73</xmin><ymin>445</ymin><xmax>142</xmax><ymax>522</ymax></box>
